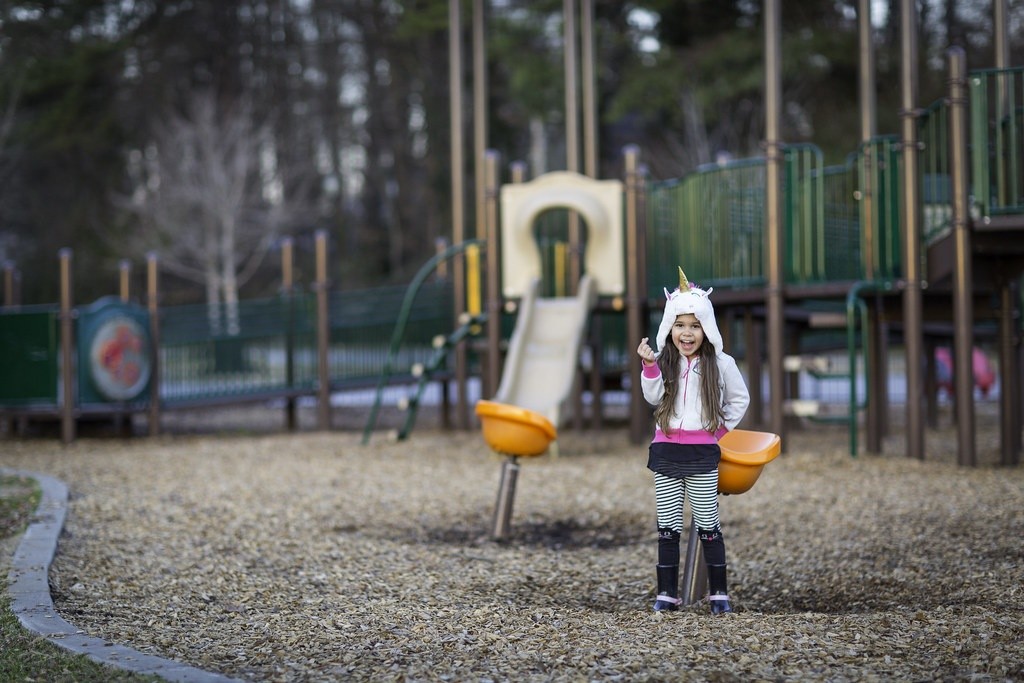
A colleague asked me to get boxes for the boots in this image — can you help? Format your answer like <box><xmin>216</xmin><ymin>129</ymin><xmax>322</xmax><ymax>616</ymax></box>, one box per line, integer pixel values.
<box><xmin>708</xmin><ymin>564</ymin><xmax>730</xmax><ymax>613</ymax></box>
<box><xmin>654</xmin><ymin>564</ymin><xmax>681</xmax><ymax>610</ymax></box>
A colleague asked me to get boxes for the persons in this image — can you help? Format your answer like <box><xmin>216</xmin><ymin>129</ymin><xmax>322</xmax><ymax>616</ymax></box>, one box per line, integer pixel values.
<box><xmin>637</xmin><ymin>266</ymin><xmax>750</xmax><ymax>615</ymax></box>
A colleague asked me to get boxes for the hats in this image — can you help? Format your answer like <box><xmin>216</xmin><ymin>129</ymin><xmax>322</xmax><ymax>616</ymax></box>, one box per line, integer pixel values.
<box><xmin>655</xmin><ymin>266</ymin><xmax>724</xmax><ymax>356</ymax></box>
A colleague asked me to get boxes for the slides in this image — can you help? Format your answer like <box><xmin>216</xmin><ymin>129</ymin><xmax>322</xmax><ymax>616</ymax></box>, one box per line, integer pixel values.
<box><xmin>490</xmin><ymin>274</ymin><xmax>598</xmax><ymax>429</ymax></box>
